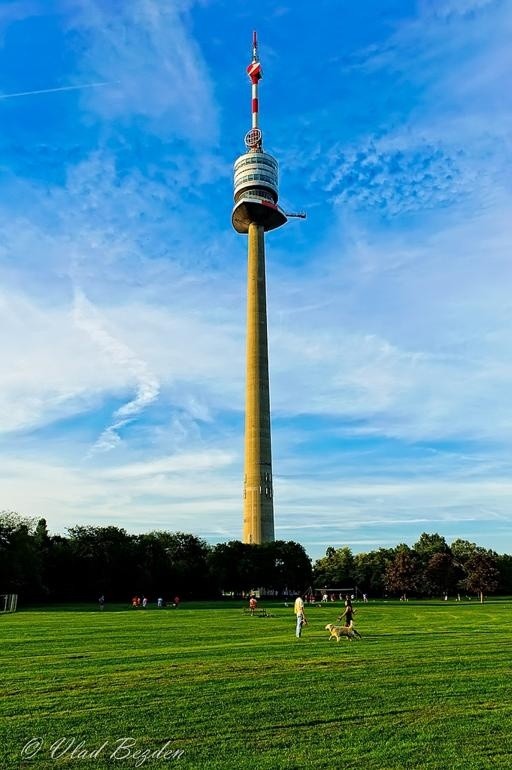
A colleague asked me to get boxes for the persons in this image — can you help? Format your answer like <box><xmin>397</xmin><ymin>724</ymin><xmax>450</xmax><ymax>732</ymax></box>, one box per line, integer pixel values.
<box><xmin>399</xmin><ymin>593</ymin><xmax>407</xmax><ymax>602</ymax></box>
<box><xmin>174</xmin><ymin>594</ymin><xmax>180</xmax><ymax>607</ymax></box>
<box><xmin>458</xmin><ymin>591</ymin><xmax>461</xmax><ymax>601</ymax></box>
<box><xmin>303</xmin><ymin>591</ymin><xmax>356</xmax><ymax>604</ymax></box>
<box><xmin>363</xmin><ymin>592</ymin><xmax>368</xmax><ymax>603</ymax></box>
<box><xmin>444</xmin><ymin>591</ymin><xmax>448</xmax><ymax>601</ymax></box>
<box><xmin>249</xmin><ymin>594</ymin><xmax>257</xmax><ymax>616</ymax></box>
<box><xmin>338</xmin><ymin>600</ymin><xmax>361</xmax><ymax>639</ymax></box>
<box><xmin>284</xmin><ymin>597</ymin><xmax>288</xmax><ymax>607</ymax></box>
<box><xmin>128</xmin><ymin>593</ymin><xmax>148</xmax><ymax>609</ymax></box>
<box><xmin>157</xmin><ymin>596</ymin><xmax>163</xmax><ymax>608</ymax></box>
<box><xmin>98</xmin><ymin>595</ymin><xmax>104</xmax><ymax>612</ymax></box>
<box><xmin>294</xmin><ymin>590</ymin><xmax>305</xmax><ymax>638</ymax></box>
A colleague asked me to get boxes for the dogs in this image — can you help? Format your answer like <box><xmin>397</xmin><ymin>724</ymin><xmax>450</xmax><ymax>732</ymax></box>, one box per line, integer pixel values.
<box><xmin>325</xmin><ymin>620</ymin><xmax>360</xmax><ymax>642</ymax></box>
